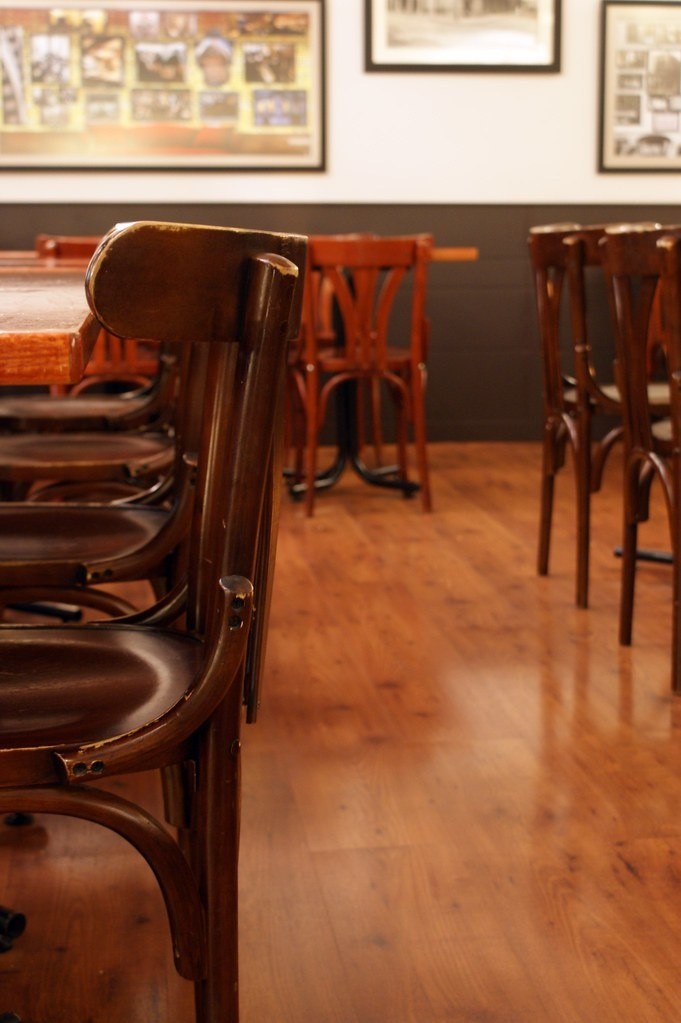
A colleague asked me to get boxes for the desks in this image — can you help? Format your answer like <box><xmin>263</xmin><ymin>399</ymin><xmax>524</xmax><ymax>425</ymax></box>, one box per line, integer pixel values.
<box><xmin>1</xmin><ymin>248</ymin><xmax>196</xmax><ymax>405</ymax></box>
<box><xmin>278</xmin><ymin>239</ymin><xmax>482</xmax><ymax>498</ymax></box>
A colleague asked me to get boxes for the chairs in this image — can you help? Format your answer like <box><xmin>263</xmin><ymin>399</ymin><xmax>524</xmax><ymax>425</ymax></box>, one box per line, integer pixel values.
<box><xmin>1</xmin><ymin>214</ymin><xmax>432</xmax><ymax>1021</ymax></box>
<box><xmin>526</xmin><ymin>220</ymin><xmax>680</xmax><ymax>697</ymax></box>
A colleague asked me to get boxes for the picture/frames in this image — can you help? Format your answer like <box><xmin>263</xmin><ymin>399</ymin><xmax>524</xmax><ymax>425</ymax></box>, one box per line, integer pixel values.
<box><xmin>595</xmin><ymin>0</ymin><xmax>680</xmax><ymax>173</ymax></box>
<box><xmin>1</xmin><ymin>0</ymin><xmax>327</xmax><ymax>171</ymax></box>
<box><xmin>365</xmin><ymin>2</ymin><xmax>564</xmax><ymax>72</ymax></box>
